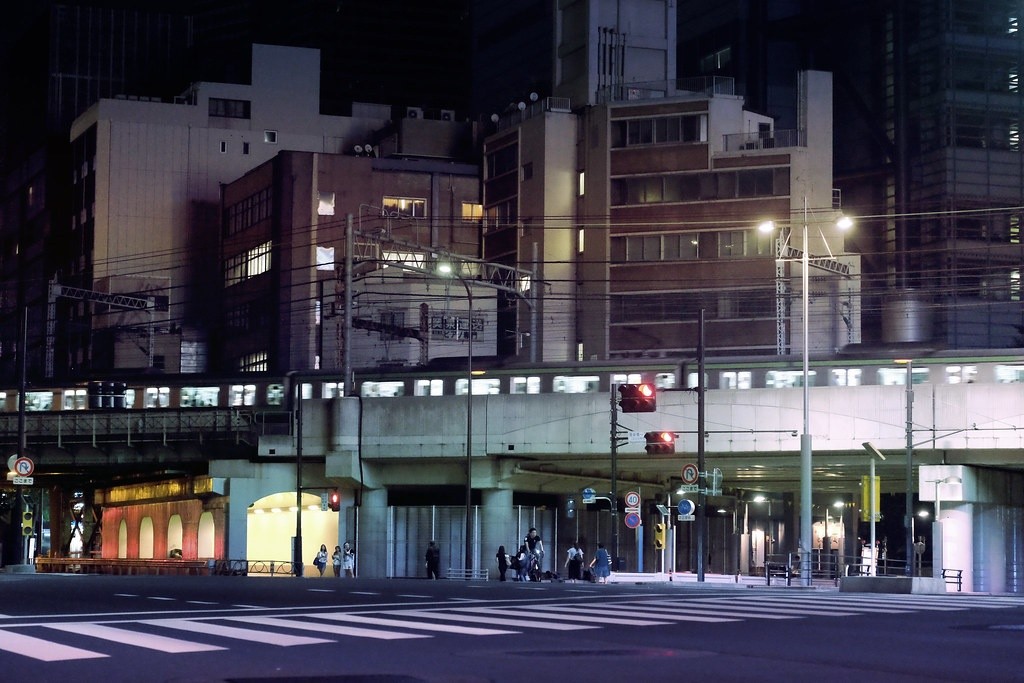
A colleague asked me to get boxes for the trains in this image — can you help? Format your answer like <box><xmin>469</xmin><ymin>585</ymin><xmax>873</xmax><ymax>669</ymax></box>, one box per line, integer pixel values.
<box><xmin>0</xmin><ymin>341</ymin><xmax>1024</xmax><ymax>416</ymax></box>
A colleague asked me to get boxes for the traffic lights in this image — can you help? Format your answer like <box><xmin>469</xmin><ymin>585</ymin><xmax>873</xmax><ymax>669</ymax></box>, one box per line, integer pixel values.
<box><xmin>654</xmin><ymin>523</ymin><xmax>665</xmax><ymax>549</ymax></box>
<box><xmin>331</xmin><ymin>492</ymin><xmax>340</xmax><ymax>512</ymax></box>
<box><xmin>642</xmin><ymin>432</ymin><xmax>675</xmax><ymax>453</ymax></box>
<box><xmin>23</xmin><ymin>512</ymin><xmax>33</xmax><ymax>535</ymax></box>
<box><xmin>618</xmin><ymin>383</ymin><xmax>656</xmax><ymax>413</ymax></box>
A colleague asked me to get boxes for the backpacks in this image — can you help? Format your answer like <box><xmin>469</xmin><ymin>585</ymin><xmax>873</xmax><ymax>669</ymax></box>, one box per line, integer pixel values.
<box><xmin>573</xmin><ymin>553</ymin><xmax>583</xmax><ymax>565</ymax></box>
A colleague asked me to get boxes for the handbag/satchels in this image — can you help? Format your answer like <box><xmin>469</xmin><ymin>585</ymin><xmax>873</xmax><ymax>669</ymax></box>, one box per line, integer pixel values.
<box><xmin>313</xmin><ymin>557</ymin><xmax>319</xmax><ymax>565</ymax></box>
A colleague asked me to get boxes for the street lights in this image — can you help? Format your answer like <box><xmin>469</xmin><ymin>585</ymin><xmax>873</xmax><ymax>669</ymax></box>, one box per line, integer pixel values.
<box><xmin>752</xmin><ymin>214</ymin><xmax>857</xmax><ymax>585</ymax></box>
<box><xmin>436</xmin><ymin>264</ymin><xmax>476</xmax><ymax>580</ymax></box>
<box><xmin>931</xmin><ymin>476</ymin><xmax>962</xmax><ymax>575</ymax></box>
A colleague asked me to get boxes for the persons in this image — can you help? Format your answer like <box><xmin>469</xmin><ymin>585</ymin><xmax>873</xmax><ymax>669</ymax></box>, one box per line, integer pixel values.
<box><xmin>424</xmin><ymin>540</ymin><xmax>440</xmax><ymax>580</ymax></box>
<box><xmin>343</xmin><ymin>541</ymin><xmax>355</xmax><ymax>578</ymax></box>
<box><xmin>522</xmin><ymin>527</ymin><xmax>545</xmax><ymax>581</ymax></box>
<box><xmin>314</xmin><ymin>544</ymin><xmax>329</xmax><ymax>577</ymax></box>
<box><xmin>564</xmin><ymin>540</ymin><xmax>585</xmax><ymax>585</ymax></box>
<box><xmin>495</xmin><ymin>545</ymin><xmax>511</xmax><ymax>583</ymax></box>
<box><xmin>331</xmin><ymin>545</ymin><xmax>343</xmax><ymax>577</ymax></box>
<box><xmin>514</xmin><ymin>544</ymin><xmax>525</xmax><ymax>581</ymax></box>
<box><xmin>589</xmin><ymin>543</ymin><xmax>612</xmax><ymax>584</ymax></box>
<box><xmin>788</xmin><ymin>555</ymin><xmax>802</xmax><ymax>588</ymax></box>
